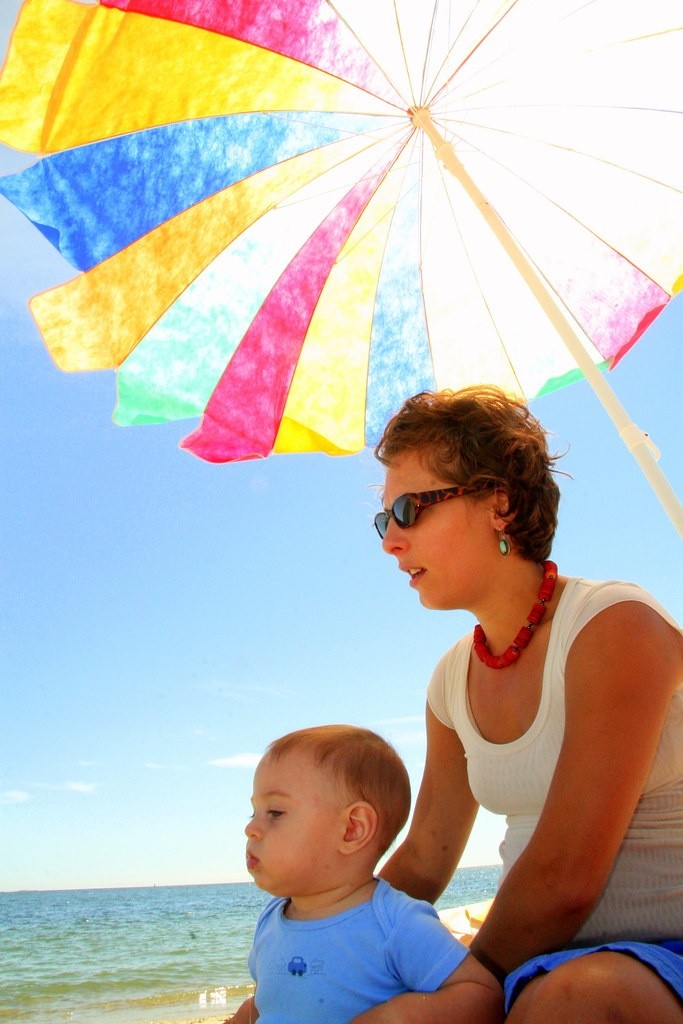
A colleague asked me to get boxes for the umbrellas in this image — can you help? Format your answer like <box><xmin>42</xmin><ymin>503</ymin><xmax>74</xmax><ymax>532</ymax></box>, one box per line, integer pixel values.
<box><xmin>0</xmin><ymin>0</ymin><xmax>683</xmax><ymax>554</ymax></box>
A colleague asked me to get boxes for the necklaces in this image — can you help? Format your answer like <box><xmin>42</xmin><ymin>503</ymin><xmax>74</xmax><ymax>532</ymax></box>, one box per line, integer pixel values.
<box><xmin>473</xmin><ymin>560</ymin><xmax>558</xmax><ymax>670</ymax></box>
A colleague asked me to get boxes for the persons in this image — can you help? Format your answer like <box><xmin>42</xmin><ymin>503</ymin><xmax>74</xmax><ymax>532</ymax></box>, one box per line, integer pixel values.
<box><xmin>371</xmin><ymin>385</ymin><xmax>683</xmax><ymax>1024</ymax></box>
<box><xmin>224</xmin><ymin>725</ymin><xmax>502</xmax><ymax>1024</ymax></box>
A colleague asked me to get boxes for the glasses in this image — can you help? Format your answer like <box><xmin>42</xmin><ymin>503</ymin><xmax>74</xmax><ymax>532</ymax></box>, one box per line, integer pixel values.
<box><xmin>375</xmin><ymin>479</ymin><xmax>500</xmax><ymax>539</ymax></box>
